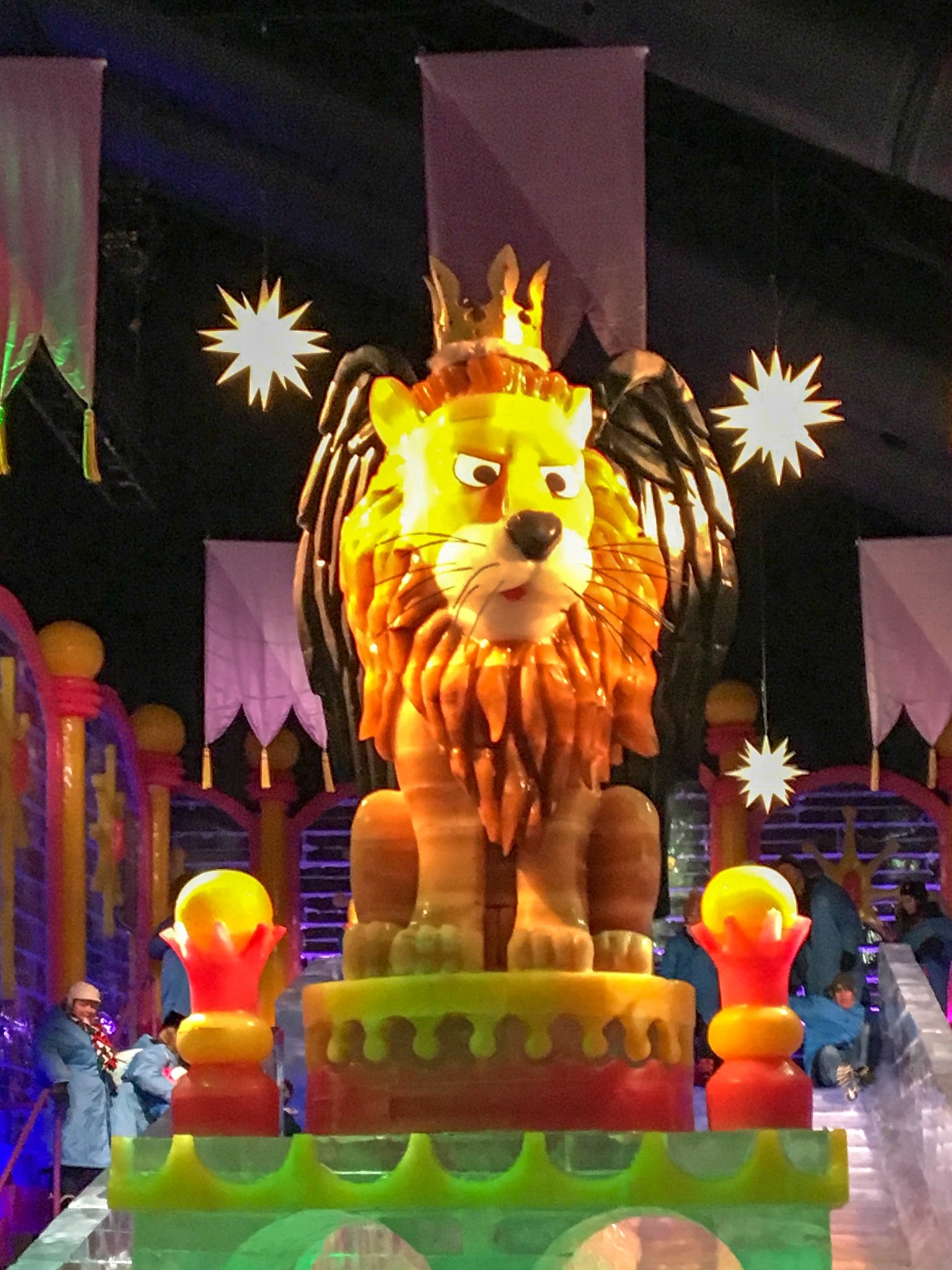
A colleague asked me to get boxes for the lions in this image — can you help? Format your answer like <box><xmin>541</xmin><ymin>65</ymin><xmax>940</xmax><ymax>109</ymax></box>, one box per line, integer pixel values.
<box><xmin>296</xmin><ymin>335</ymin><xmax>740</xmax><ymax>974</ymax></box>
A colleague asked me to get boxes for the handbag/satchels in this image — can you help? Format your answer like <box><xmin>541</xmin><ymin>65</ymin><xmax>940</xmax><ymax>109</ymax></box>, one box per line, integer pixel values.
<box><xmin>136</xmin><ymin>1091</ymin><xmax>172</xmax><ymax>1125</ymax></box>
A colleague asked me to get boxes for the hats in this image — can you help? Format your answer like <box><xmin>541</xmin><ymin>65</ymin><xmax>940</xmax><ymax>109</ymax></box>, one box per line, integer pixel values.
<box><xmin>67</xmin><ymin>981</ymin><xmax>101</xmax><ymax>1008</ymax></box>
<box><xmin>830</xmin><ymin>972</ymin><xmax>858</xmax><ymax>998</ymax></box>
<box><xmin>900</xmin><ymin>881</ymin><xmax>930</xmax><ymax>901</ymax></box>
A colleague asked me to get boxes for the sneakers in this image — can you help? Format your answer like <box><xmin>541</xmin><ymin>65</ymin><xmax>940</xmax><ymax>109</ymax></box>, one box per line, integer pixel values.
<box><xmin>837</xmin><ymin>1062</ymin><xmax>859</xmax><ymax>1103</ymax></box>
<box><xmin>857</xmin><ymin>1069</ymin><xmax>875</xmax><ymax>1087</ymax></box>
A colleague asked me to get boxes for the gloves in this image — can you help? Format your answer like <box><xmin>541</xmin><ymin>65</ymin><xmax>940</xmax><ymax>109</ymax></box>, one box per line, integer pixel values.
<box><xmin>55</xmin><ymin>1083</ymin><xmax>70</xmax><ymax>1102</ymax></box>
<box><xmin>102</xmin><ymin>1070</ymin><xmax>117</xmax><ymax>1097</ymax></box>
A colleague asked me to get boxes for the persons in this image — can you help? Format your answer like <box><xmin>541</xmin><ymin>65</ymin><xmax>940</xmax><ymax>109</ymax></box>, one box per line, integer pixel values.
<box><xmin>652</xmin><ymin>844</ymin><xmax>952</xmax><ymax>1102</ymax></box>
<box><xmin>148</xmin><ymin>873</ymin><xmax>197</xmax><ymax>1027</ymax></box>
<box><xmin>36</xmin><ymin>982</ymin><xmax>303</xmax><ymax>1212</ymax></box>
